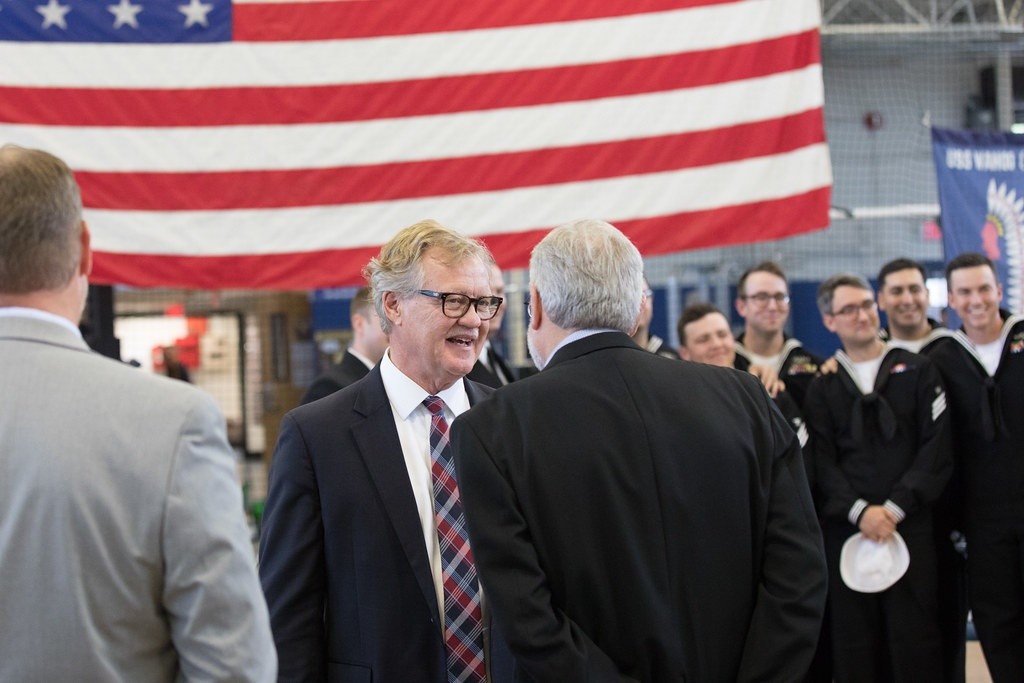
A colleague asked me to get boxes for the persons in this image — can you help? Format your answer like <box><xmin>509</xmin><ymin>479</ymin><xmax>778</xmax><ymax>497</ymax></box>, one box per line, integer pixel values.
<box><xmin>255</xmin><ymin>216</ymin><xmax>1024</xmax><ymax>683</ymax></box>
<box><xmin>1</xmin><ymin>143</ymin><xmax>279</xmax><ymax>683</ymax></box>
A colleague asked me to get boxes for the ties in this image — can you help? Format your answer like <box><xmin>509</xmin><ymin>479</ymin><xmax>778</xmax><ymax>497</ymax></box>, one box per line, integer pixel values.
<box><xmin>836</xmin><ymin>352</ymin><xmax>897</xmax><ymax>441</ymax></box>
<box><xmin>960</xmin><ymin>337</ymin><xmax>1006</xmax><ymax>439</ymax></box>
<box><xmin>487</xmin><ymin>347</ymin><xmax>503</xmax><ymax>385</ymax></box>
<box><xmin>422</xmin><ymin>396</ymin><xmax>488</xmax><ymax>683</ymax></box>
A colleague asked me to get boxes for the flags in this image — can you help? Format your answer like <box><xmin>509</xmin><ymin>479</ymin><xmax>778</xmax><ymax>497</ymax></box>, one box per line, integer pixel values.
<box><xmin>0</xmin><ymin>0</ymin><xmax>834</xmax><ymax>290</ymax></box>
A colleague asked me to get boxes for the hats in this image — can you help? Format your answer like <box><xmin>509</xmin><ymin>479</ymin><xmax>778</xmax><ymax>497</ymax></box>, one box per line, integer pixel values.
<box><xmin>839</xmin><ymin>530</ymin><xmax>911</xmax><ymax>593</ymax></box>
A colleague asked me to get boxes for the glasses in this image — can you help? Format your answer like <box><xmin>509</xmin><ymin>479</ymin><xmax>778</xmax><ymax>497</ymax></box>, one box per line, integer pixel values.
<box><xmin>414</xmin><ymin>289</ymin><xmax>504</xmax><ymax>321</ymax></box>
<box><xmin>826</xmin><ymin>300</ymin><xmax>873</xmax><ymax>316</ymax></box>
<box><xmin>744</xmin><ymin>292</ymin><xmax>790</xmax><ymax>306</ymax></box>
